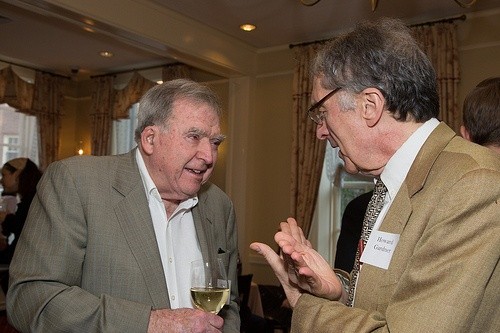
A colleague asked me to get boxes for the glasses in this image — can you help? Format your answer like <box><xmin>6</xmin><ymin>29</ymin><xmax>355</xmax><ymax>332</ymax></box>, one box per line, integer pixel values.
<box><xmin>305</xmin><ymin>86</ymin><xmax>342</xmax><ymax>125</ymax></box>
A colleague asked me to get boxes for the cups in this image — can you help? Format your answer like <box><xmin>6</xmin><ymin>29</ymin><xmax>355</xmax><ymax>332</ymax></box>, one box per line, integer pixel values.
<box><xmin>190</xmin><ymin>257</ymin><xmax>230</xmax><ymax>316</ymax></box>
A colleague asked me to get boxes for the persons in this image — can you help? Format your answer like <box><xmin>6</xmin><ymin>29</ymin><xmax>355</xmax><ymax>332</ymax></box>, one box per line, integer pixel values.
<box><xmin>6</xmin><ymin>79</ymin><xmax>241</xmax><ymax>333</ymax></box>
<box><xmin>249</xmin><ymin>18</ymin><xmax>500</xmax><ymax>333</ymax></box>
<box><xmin>0</xmin><ymin>158</ymin><xmax>43</xmax><ymax>296</ymax></box>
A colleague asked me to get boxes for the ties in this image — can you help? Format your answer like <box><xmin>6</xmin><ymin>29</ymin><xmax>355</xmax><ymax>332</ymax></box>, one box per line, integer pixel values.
<box><xmin>345</xmin><ymin>177</ymin><xmax>389</xmax><ymax>307</ymax></box>
